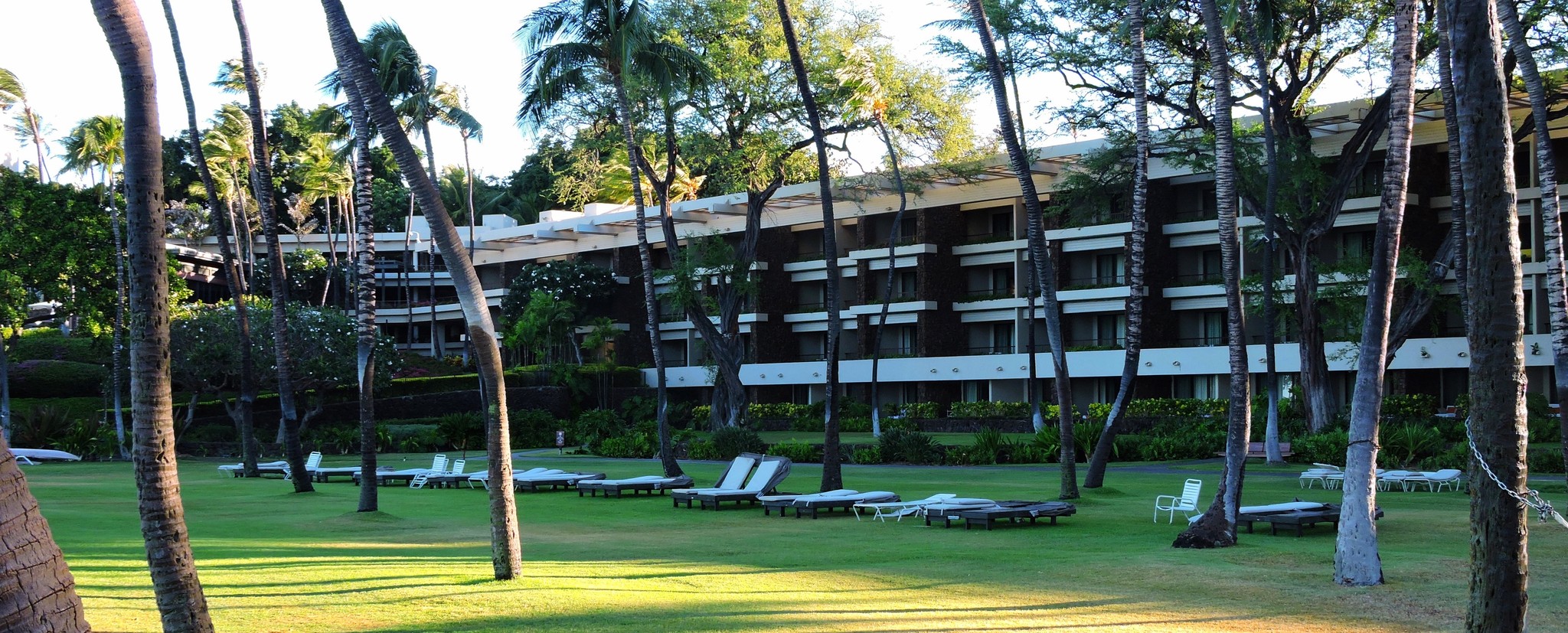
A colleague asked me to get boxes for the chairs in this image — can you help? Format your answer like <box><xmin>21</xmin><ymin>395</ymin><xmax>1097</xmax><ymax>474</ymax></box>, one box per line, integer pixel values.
<box><xmin>1154</xmin><ymin>479</ymin><xmax>1202</xmax><ymax>524</ymax></box>
<box><xmin>1238</xmin><ymin>496</ymin><xmax>1342</xmax><ymax>537</ymax></box>
<box><xmin>218</xmin><ymin>451</ymin><xmax>1077</xmax><ymax>531</ymax></box>
<box><xmin>1299</xmin><ymin>462</ymin><xmax>1461</xmax><ymax>493</ymax></box>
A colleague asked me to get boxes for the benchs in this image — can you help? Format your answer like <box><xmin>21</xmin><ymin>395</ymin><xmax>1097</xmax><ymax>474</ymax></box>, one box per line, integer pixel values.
<box><xmin>1247</xmin><ymin>442</ymin><xmax>1295</xmax><ymax>463</ymax></box>
